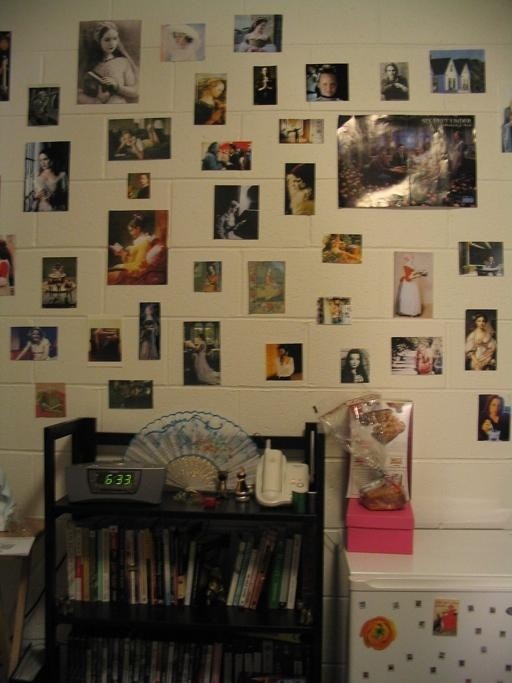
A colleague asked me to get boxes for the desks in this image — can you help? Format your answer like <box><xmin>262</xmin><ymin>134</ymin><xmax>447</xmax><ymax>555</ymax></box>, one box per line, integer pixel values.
<box><xmin>0</xmin><ymin>516</ymin><xmax>43</xmax><ymax>683</ymax></box>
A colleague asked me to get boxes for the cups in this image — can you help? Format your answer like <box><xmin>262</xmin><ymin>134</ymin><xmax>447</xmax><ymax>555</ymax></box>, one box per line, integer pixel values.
<box><xmin>292</xmin><ymin>490</ymin><xmax>306</xmax><ymax>513</ymax></box>
<box><xmin>306</xmin><ymin>492</ymin><xmax>320</xmax><ymax>513</ymax></box>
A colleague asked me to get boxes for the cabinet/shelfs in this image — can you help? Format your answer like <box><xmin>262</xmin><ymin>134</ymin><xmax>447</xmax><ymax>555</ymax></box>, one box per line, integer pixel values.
<box><xmin>37</xmin><ymin>416</ymin><xmax>325</xmax><ymax>682</ymax></box>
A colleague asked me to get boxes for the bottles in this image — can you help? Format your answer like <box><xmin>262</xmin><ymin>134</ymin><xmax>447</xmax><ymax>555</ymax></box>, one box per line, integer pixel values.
<box><xmin>236</xmin><ymin>473</ymin><xmax>248</xmax><ymax>502</ymax></box>
<box><xmin>217</xmin><ymin>471</ymin><xmax>228</xmax><ymax>498</ymax></box>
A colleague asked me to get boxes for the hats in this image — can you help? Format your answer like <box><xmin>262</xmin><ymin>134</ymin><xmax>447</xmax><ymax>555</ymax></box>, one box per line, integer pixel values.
<box><xmin>169</xmin><ymin>24</ymin><xmax>200</xmax><ymax>51</ymax></box>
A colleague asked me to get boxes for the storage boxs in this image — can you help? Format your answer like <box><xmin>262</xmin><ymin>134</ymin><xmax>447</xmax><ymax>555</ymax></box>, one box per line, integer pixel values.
<box><xmin>343</xmin><ymin>498</ymin><xmax>415</xmax><ymax>555</ymax></box>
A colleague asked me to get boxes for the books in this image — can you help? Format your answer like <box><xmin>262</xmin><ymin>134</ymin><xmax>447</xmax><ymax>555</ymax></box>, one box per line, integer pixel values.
<box><xmin>65</xmin><ymin>631</ymin><xmax>304</xmax><ymax>682</ymax></box>
<box><xmin>66</xmin><ymin>516</ymin><xmax>302</xmax><ymax>609</ymax></box>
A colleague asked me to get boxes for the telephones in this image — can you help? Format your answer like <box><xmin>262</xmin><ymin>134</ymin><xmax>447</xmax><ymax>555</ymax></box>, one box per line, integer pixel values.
<box><xmin>255</xmin><ymin>440</ymin><xmax>310</xmax><ymax>508</ymax></box>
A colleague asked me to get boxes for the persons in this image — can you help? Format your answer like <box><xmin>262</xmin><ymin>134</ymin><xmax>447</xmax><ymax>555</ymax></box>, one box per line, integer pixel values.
<box><xmin>0</xmin><ymin>15</ymin><xmax>511</xmax><ymax>438</ymax></box>
